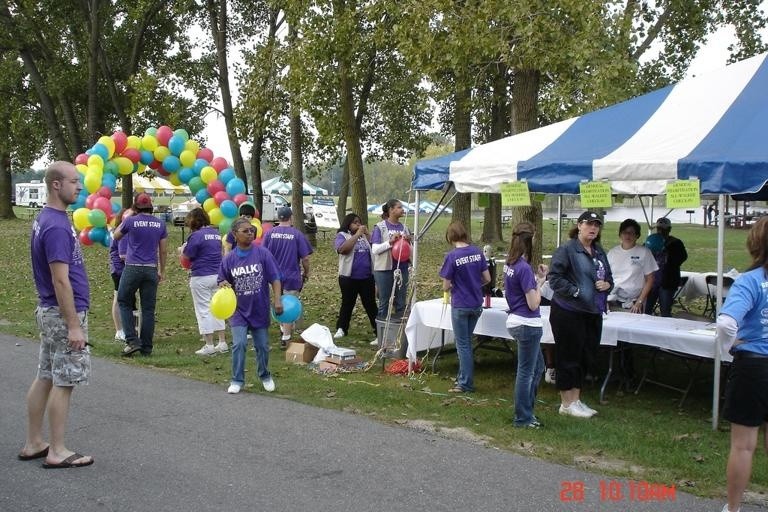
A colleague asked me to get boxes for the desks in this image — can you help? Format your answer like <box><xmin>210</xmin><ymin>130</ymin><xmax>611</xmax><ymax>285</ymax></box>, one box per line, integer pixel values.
<box><xmin>26</xmin><ymin>207</ymin><xmax>39</xmax><ymax>222</ymax></box>
<box><xmin>406</xmin><ymin>293</ymin><xmax>741</xmax><ymax>422</ymax></box>
<box><xmin>63</xmin><ymin>209</ymin><xmax>74</xmax><ymax>220</ymax></box>
<box><xmin>672</xmin><ymin>267</ymin><xmax>740</xmax><ymax>301</ymax></box>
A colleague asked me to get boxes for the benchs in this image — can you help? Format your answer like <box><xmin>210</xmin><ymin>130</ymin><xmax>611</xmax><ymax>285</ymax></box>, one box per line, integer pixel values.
<box><xmin>707</xmin><ymin>212</ymin><xmax>747</xmax><ymax>228</ymax></box>
<box><xmin>478</xmin><ymin>211</ymin><xmax>582</xmax><ymax>231</ymax></box>
<box><xmin>18</xmin><ymin>212</ymin><xmax>26</xmax><ymax>220</ymax></box>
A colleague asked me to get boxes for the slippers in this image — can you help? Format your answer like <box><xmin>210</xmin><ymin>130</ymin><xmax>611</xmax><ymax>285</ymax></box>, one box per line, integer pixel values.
<box><xmin>18</xmin><ymin>443</ymin><xmax>95</xmax><ymax>469</ymax></box>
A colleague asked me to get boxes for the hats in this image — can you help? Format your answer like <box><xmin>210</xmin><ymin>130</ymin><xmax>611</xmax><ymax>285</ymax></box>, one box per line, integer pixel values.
<box><xmin>133</xmin><ymin>193</ymin><xmax>152</xmax><ymax>207</ymax></box>
<box><xmin>651</xmin><ymin>216</ymin><xmax>672</xmax><ymax>229</ymax></box>
<box><xmin>277</xmin><ymin>207</ymin><xmax>292</xmax><ymax>219</ymax></box>
<box><xmin>575</xmin><ymin>211</ymin><xmax>603</xmax><ymax>226</ymax></box>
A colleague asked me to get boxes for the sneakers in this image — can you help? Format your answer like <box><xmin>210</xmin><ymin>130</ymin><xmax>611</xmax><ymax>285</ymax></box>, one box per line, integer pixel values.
<box><xmin>334</xmin><ymin>327</ymin><xmax>348</xmax><ymax>343</ymax></box>
<box><xmin>226</xmin><ymin>380</ymin><xmax>243</xmax><ymax>394</ymax></box>
<box><xmin>277</xmin><ymin>327</ymin><xmax>295</xmax><ymax>347</ymax></box>
<box><xmin>510</xmin><ymin>413</ymin><xmax>547</xmax><ymax>434</ymax></box>
<box><xmin>194</xmin><ymin>341</ymin><xmax>231</xmax><ymax>358</ymax></box>
<box><xmin>111</xmin><ymin>327</ymin><xmax>159</xmax><ymax>357</ymax></box>
<box><xmin>258</xmin><ymin>372</ymin><xmax>276</xmax><ymax>393</ymax></box>
<box><xmin>558</xmin><ymin>399</ymin><xmax>598</xmax><ymax>420</ymax></box>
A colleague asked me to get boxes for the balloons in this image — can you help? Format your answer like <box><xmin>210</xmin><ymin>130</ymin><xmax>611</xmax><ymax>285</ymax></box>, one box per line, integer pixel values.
<box><xmin>70</xmin><ymin>127</ymin><xmax>261</xmax><ymax>247</ymax></box>
<box><xmin>272</xmin><ymin>295</ymin><xmax>302</xmax><ymax>323</ymax></box>
<box><xmin>210</xmin><ymin>286</ymin><xmax>236</xmax><ymax>320</ymax></box>
<box><xmin>392</xmin><ymin>238</ymin><xmax>410</xmax><ymax>261</ymax></box>
<box><xmin>179</xmin><ymin>254</ymin><xmax>191</xmax><ymax>269</ymax></box>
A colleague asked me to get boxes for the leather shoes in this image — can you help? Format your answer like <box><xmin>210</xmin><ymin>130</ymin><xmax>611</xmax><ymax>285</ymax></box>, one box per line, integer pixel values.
<box><xmin>448</xmin><ymin>381</ymin><xmax>479</xmax><ymax>393</ymax></box>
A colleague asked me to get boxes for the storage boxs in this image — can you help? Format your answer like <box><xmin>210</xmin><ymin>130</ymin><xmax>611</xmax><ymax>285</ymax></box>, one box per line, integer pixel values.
<box><xmin>319</xmin><ymin>358</ymin><xmax>362</xmax><ymax>371</ymax></box>
<box><xmin>285</xmin><ymin>338</ymin><xmax>317</xmax><ymax>364</ymax></box>
<box><xmin>324</xmin><ymin>349</ymin><xmax>356</xmax><ymax>365</ymax></box>
<box><xmin>375</xmin><ymin>310</ymin><xmax>412</xmax><ymax>360</ymax></box>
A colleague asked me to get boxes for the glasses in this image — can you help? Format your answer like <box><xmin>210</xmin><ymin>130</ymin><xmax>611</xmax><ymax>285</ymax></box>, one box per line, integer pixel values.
<box><xmin>233</xmin><ymin>228</ymin><xmax>256</xmax><ymax>234</ymax></box>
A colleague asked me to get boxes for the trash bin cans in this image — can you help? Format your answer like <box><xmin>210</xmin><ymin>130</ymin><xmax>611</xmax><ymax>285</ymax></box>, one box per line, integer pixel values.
<box><xmin>375</xmin><ymin>312</ymin><xmax>425</xmax><ymax>358</ymax></box>
<box><xmin>304</xmin><ymin>222</ymin><xmax>317</xmax><ymax>246</ymax></box>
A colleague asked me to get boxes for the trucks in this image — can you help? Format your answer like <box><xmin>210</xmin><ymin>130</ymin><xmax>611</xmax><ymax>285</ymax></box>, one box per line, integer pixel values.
<box><xmin>172</xmin><ymin>190</ymin><xmax>293</xmax><ymax>223</ymax></box>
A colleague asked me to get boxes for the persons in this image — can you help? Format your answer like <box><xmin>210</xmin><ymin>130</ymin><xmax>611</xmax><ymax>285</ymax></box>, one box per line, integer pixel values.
<box><xmin>716</xmin><ymin>217</ymin><xmax>768</xmax><ymax>512</ymax></box>
<box><xmin>605</xmin><ymin>218</ymin><xmax>660</xmax><ymax>391</ymax></box>
<box><xmin>114</xmin><ymin>195</ymin><xmax>168</xmax><ymax>355</ymax></box>
<box><xmin>109</xmin><ymin>209</ymin><xmax>136</xmax><ymax>341</ymax></box>
<box><xmin>543</xmin><ymin>212</ymin><xmax>615</xmax><ymax>417</ymax></box>
<box><xmin>181</xmin><ymin>208</ymin><xmax>229</xmax><ymax>354</ymax></box>
<box><xmin>262</xmin><ymin>207</ymin><xmax>312</xmax><ymax>348</ymax></box>
<box><xmin>437</xmin><ymin>224</ymin><xmax>491</xmax><ymax>392</ymax></box>
<box><xmin>226</xmin><ymin>204</ymin><xmax>256</xmax><ymax>339</ymax></box>
<box><xmin>334</xmin><ymin>213</ymin><xmax>378</xmax><ymax>337</ymax></box>
<box><xmin>370</xmin><ymin>199</ymin><xmax>413</xmax><ymax>345</ymax></box>
<box><xmin>645</xmin><ymin>218</ymin><xmax>688</xmax><ymax>317</ymax></box>
<box><xmin>503</xmin><ymin>222</ymin><xmax>549</xmax><ymax>428</ymax></box>
<box><xmin>18</xmin><ymin>159</ymin><xmax>96</xmax><ymax>470</ymax></box>
<box><xmin>217</xmin><ymin>218</ymin><xmax>283</xmax><ymax>393</ymax></box>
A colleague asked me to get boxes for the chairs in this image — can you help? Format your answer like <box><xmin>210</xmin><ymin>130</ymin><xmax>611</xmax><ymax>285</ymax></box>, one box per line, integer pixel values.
<box><xmin>651</xmin><ymin>275</ymin><xmax>688</xmax><ymax>314</ymax></box>
<box><xmin>704</xmin><ymin>275</ymin><xmax>734</xmax><ymax>319</ymax></box>
